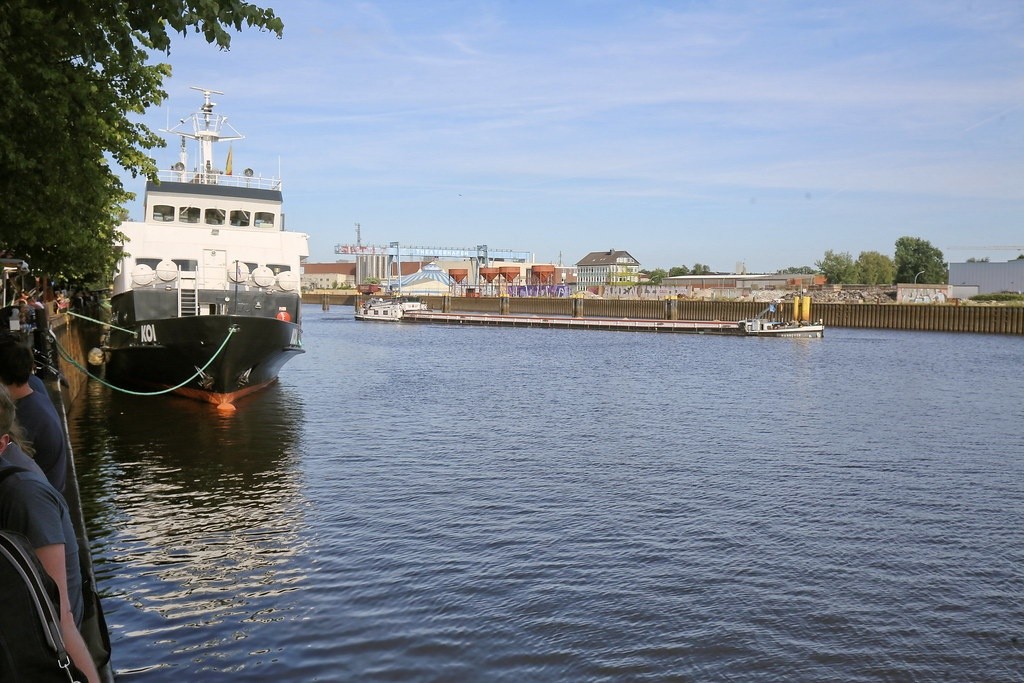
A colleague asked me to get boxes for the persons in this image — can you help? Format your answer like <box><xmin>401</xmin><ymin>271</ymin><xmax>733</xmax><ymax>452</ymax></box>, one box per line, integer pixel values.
<box><xmin>0</xmin><ymin>329</ymin><xmax>112</xmax><ymax>683</ymax></box>
<box><xmin>53</xmin><ymin>291</ymin><xmax>70</xmax><ymax>314</ymax></box>
<box><xmin>14</xmin><ymin>290</ymin><xmax>45</xmax><ymax>327</ymax></box>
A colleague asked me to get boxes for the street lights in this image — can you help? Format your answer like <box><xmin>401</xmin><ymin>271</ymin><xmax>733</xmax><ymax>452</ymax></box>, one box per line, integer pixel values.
<box><xmin>913</xmin><ymin>270</ymin><xmax>925</xmax><ymax>298</ymax></box>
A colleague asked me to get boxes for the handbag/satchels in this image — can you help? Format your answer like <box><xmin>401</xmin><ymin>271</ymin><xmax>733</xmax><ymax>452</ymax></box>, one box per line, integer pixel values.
<box><xmin>81</xmin><ymin>591</ymin><xmax>111</xmax><ymax>670</ymax></box>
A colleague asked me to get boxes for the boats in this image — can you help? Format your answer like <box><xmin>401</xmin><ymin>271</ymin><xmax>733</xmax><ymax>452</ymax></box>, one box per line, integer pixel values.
<box><xmin>352</xmin><ymin>296</ymin><xmax>402</xmax><ymax>322</ymax></box>
<box><xmin>100</xmin><ymin>85</ymin><xmax>311</xmax><ymax>420</ymax></box>
